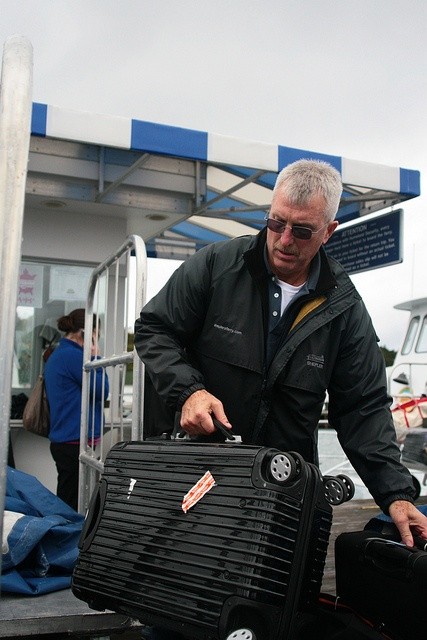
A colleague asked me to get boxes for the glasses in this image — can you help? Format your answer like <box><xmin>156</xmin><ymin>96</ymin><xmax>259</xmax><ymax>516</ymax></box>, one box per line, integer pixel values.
<box><xmin>262</xmin><ymin>210</ymin><xmax>327</xmax><ymax>240</ymax></box>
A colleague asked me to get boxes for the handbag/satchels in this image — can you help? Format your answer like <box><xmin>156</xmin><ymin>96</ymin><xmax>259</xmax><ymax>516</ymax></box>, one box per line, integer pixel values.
<box><xmin>22</xmin><ymin>378</ymin><xmax>49</xmax><ymax>438</ymax></box>
<box><xmin>390</xmin><ymin>398</ymin><xmax>426</xmax><ymax>443</ymax></box>
<box><xmin>334</xmin><ymin>518</ymin><xmax>426</xmax><ymax>639</ymax></box>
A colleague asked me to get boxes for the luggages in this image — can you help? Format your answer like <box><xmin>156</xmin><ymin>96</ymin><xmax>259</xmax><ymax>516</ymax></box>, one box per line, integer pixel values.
<box><xmin>70</xmin><ymin>417</ymin><xmax>354</xmax><ymax>640</ymax></box>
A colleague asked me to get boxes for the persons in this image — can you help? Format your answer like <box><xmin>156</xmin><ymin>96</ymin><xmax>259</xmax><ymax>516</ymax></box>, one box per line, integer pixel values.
<box><xmin>41</xmin><ymin>307</ymin><xmax>108</xmax><ymax>509</ymax></box>
<box><xmin>135</xmin><ymin>160</ymin><xmax>426</xmax><ymax>549</ymax></box>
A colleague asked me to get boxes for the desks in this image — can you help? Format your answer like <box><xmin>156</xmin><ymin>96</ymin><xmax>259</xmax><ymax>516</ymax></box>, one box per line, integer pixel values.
<box><xmin>10</xmin><ymin>417</ymin><xmax>134</xmax><ymax>428</ymax></box>
